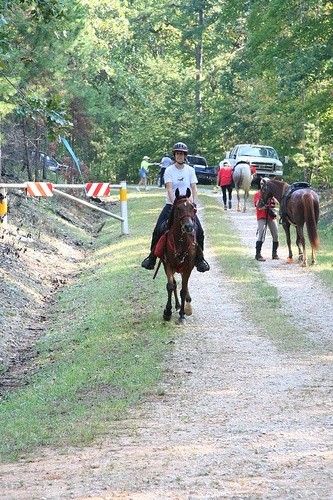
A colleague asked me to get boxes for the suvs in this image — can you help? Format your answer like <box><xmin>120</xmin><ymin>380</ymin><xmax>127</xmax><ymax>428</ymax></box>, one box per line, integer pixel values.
<box><xmin>218</xmin><ymin>144</ymin><xmax>283</xmax><ymax>192</ymax></box>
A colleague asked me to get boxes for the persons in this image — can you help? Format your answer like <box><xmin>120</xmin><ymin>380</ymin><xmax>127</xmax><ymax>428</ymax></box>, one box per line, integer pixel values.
<box><xmin>254</xmin><ymin>177</ymin><xmax>281</xmax><ymax>261</ymax></box>
<box><xmin>159</xmin><ymin>154</ymin><xmax>175</xmax><ymax>188</ymax></box>
<box><xmin>136</xmin><ymin>156</ymin><xmax>160</xmax><ymax>191</ymax></box>
<box><xmin>216</xmin><ymin>162</ymin><xmax>234</xmax><ymax>210</ymax></box>
<box><xmin>142</xmin><ymin>142</ymin><xmax>210</xmax><ymax>273</ymax></box>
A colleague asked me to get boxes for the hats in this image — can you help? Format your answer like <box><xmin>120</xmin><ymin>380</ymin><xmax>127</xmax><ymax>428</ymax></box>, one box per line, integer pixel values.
<box><xmin>142</xmin><ymin>156</ymin><xmax>150</xmax><ymax>160</ymax></box>
<box><xmin>224</xmin><ymin>162</ymin><xmax>228</xmax><ymax>165</ymax></box>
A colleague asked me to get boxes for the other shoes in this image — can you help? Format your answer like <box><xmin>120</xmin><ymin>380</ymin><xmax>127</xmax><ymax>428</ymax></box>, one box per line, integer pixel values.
<box><xmin>229</xmin><ymin>204</ymin><xmax>232</xmax><ymax>209</ymax></box>
<box><xmin>224</xmin><ymin>206</ymin><xmax>227</xmax><ymax>210</ymax></box>
<box><xmin>145</xmin><ymin>187</ymin><xmax>150</xmax><ymax>191</ymax></box>
<box><xmin>136</xmin><ymin>186</ymin><xmax>140</xmax><ymax>191</ymax></box>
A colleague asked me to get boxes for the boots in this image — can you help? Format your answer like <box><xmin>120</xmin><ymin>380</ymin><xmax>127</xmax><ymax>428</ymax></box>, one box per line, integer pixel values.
<box><xmin>195</xmin><ymin>235</ymin><xmax>210</xmax><ymax>272</ymax></box>
<box><xmin>255</xmin><ymin>241</ymin><xmax>266</xmax><ymax>261</ymax></box>
<box><xmin>272</xmin><ymin>241</ymin><xmax>280</xmax><ymax>260</ymax></box>
<box><xmin>142</xmin><ymin>231</ymin><xmax>160</xmax><ymax>268</ymax></box>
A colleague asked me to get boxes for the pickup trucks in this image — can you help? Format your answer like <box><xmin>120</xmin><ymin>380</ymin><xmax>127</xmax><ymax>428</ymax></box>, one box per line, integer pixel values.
<box><xmin>157</xmin><ymin>154</ymin><xmax>218</xmax><ymax>189</ymax></box>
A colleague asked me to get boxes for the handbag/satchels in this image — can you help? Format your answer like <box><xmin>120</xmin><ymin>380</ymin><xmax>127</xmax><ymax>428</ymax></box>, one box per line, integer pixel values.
<box><xmin>139</xmin><ymin>169</ymin><xmax>146</xmax><ymax>178</ymax></box>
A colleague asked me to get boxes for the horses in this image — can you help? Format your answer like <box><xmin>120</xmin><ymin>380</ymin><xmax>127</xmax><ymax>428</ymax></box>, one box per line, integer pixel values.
<box><xmin>161</xmin><ymin>187</ymin><xmax>196</xmax><ymax>326</ymax></box>
<box><xmin>232</xmin><ymin>161</ymin><xmax>253</xmax><ymax>213</ymax></box>
<box><xmin>258</xmin><ymin>178</ymin><xmax>320</xmax><ymax>268</ymax></box>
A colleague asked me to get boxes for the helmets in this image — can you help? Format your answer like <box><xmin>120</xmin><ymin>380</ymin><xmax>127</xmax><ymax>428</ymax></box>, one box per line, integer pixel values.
<box><xmin>261</xmin><ymin>177</ymin><xmax>269</xmax><ymax>188</ymax></box>
<box><xmin>172</xmin><ymin>143</ymin><xmax>188</xmax><ymax>155</ymax></box>
